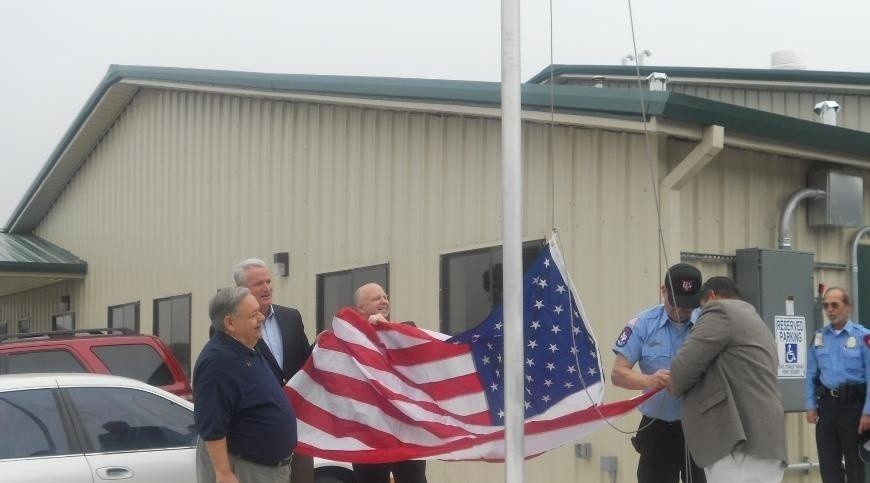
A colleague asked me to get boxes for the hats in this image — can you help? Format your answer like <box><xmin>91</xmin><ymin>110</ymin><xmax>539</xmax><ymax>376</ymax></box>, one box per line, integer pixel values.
<box><xmin>665</xmin><ymin>262</ymin><xmax>703</xmax><ymax>308</ymax></box>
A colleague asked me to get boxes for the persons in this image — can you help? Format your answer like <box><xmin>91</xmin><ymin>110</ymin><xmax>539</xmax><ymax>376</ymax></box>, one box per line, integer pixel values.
<box><xmin>192</xmin><ymin>287</ymin><xmax>297</xmax><ymax>483</ymax></box>
<box><xmin>611</xmin><ymin>264</ymin><xmax>788</xmax><ymax>483</ymax></box>
<box><xmin>804</xmin><ymin>287</ymin><xmax>870</xmax><ymax>483</ymax></box>
<box><xmin>209</xmin><ymin>258</ymin><xmax>328</xmax><ymax>383</ymax></box>
<box><xmin>351</xmin><ymin>282</ymin><xmax>427</xmax><ymax>483</ymax></box>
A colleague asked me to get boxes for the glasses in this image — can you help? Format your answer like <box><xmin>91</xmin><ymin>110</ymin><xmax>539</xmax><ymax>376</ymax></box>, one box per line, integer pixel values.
<box><xmin>822</xmin><ymin>301</ymin><xmax>846</xmax><ymax>310</ymax></box>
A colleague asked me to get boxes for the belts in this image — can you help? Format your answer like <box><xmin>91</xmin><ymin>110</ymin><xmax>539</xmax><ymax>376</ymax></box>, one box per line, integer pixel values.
<box><xmin>229</xmin><ymin>450</ymin><xmax>294</xmax><ymax>468</ymax></box>
<box><xmin>825</xmin><ymin>388</ymin><xmax>840</xmax><ymax>398</ymax></box>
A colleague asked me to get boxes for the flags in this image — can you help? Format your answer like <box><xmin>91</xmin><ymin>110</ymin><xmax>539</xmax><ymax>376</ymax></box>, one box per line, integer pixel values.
<box><xmin>282</xmin><ymin>230</ymin><xmax>665</xmax><ymax>464</ymax></box>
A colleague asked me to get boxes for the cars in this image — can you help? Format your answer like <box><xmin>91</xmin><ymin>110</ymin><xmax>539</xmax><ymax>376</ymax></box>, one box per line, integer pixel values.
<box><xmin>0</xmin><ymin>327</ymin><xmax>216</xmax><ymax>483</ymax></box>
